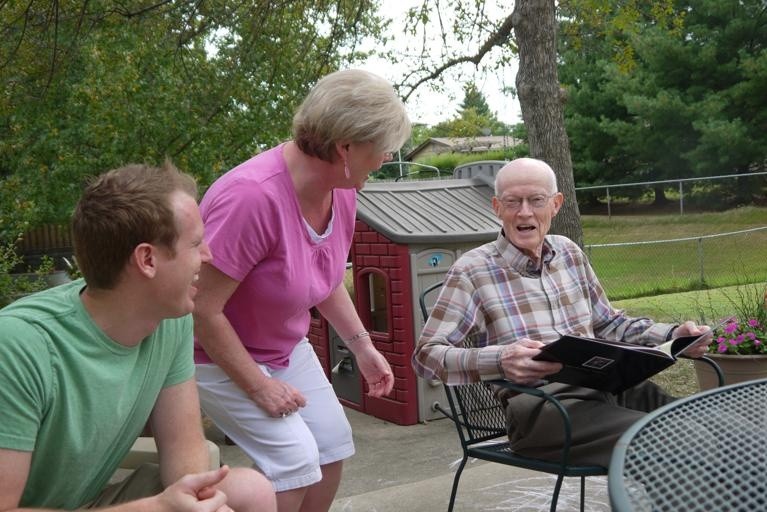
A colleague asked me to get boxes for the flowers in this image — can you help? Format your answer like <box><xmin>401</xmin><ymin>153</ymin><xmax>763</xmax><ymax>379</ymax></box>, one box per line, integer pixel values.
<box><xmin>706</xmin><ymin>312</ymin><xmax>765</xmax><ymax>354</ymax></box>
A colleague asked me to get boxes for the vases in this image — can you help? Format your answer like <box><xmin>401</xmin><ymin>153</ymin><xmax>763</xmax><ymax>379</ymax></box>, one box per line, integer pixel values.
<box><xmin>692</xmin><ymin>352</ymin><xmax>766</xmax><ymax>392</ymax></box>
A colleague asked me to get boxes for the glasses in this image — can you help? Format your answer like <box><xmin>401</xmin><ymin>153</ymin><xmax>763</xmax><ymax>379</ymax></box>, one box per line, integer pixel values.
<box><xmin>496</xmin><ymin>191</ymin><xmax>556</xmax><ymax>209</ymax></box>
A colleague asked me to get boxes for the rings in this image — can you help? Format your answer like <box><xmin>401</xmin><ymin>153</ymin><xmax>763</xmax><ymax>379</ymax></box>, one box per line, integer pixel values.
<box><xmin>281</xmin><ymin>411</ymin><xmax>289</xmax><ymax>416</ymax></box>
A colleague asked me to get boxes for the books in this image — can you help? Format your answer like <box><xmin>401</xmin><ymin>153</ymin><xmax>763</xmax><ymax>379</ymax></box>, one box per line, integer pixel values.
<box><xmin>532</xmin><ymin>312</ymin><xmax>735</xmax><ymax>396</ymax></box>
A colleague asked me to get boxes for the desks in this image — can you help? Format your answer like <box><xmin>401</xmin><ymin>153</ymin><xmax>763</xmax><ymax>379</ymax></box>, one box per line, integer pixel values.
<box><xmin>605</xmin><ymin>376</ymin><xmax>765</xmax><ymax>511</ymax></box>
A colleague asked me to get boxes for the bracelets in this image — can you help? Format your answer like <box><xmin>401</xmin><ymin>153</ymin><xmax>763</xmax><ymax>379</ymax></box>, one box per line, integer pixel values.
<box><xmin>343</xmin><ymin>331</ymin><xmax>370</xmax><ymax>345</ymax></box>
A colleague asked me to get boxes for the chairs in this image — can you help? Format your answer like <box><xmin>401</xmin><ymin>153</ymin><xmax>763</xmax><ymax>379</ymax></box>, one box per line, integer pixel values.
<box><xmin>419</xmin><ymin>271</ymin><xmax>725</xmax><ymax>511</ymax></box>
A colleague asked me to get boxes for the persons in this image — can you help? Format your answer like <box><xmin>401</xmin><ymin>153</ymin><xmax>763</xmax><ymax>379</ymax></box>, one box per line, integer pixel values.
<box><xmin>410</xmin><ymin>156</ymin><xmax>767</xmax><ymax>512</ymax></box>
<box><xmin>193</xmin><ymin>67</ymin><xmax>414</xmax><ymax>512</ymax></box>
<box><xmin>0</xmin><ymin>154</ymin><xmax>277</xmax><ymax>512</ymax></box>
